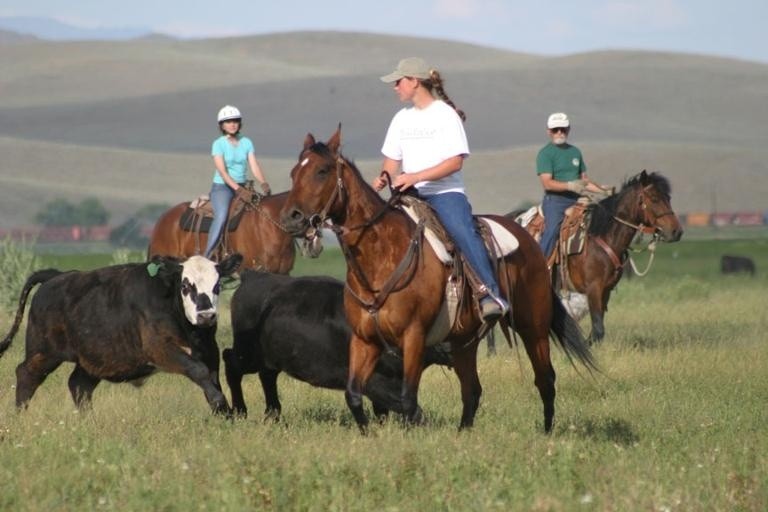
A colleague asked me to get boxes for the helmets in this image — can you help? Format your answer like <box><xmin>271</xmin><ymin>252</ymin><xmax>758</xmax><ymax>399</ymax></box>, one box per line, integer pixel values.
<box><xmin>217</xmin><ymin>106</ymin><xmax>242</xmax><ymax>124</ymax></box>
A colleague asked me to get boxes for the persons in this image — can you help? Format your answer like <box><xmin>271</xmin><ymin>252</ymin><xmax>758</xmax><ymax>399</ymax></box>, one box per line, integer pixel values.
<box><xmin>535</xmin><ymin>112</ymin><xmax>613</xmax><ymax>263</ymax></box>
<box><xmin>369</xmin><ymin>57</ymin><xmax>503</xmax><ymax>322</ymax></box>
<box><xmin>204</xmin><ymin>106</ymin><xmax>270</xmax><ymax>261</ymax></box>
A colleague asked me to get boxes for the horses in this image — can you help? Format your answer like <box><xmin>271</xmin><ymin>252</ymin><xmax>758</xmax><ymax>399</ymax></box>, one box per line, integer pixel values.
<box><xmin>486</xmin><ymin>170</ymin><xmax>684</xmax><ymax>359</ymax></box>
<box><xmin>146</xmin><ymin>192</ymin><xmax>323</xmax><ymax>276</ymax></box>
<box><xmin>278</xmin><ymin>121</ymin><xmax>616</xmax><ymax>438</ymax></box>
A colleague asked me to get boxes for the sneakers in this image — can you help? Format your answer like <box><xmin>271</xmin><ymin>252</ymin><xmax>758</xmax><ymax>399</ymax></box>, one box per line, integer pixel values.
<box><xmin>481</xmin><ymin>303</ymin><xmax>502</xmax><ymax>319</ymax></box>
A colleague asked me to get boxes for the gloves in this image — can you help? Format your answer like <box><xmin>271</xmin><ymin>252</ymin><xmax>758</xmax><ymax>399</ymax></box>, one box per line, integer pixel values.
<box><xmin>569</xmin><ymin>179</ymin><xmax>589</xmax><ymax>195</ymax></box>
<box><xmin>261</xmin><ymin>182</ymin><xmax>270</xmax><ymax>195</ymax></box>
<box><xmin>235</xmin><ymin>186</ymin><xmax>255</xmax><ymax>202</ymax></box>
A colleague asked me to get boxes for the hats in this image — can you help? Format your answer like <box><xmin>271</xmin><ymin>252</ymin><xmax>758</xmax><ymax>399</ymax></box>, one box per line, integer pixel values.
<box><xmin>547</xmin><ymin>113</ymin><xmax>570</xmax><ymax>130</ymax></box>
<box><xmin>380</xmin><ymin>57</ymin><xmax>432</xmax><ymax>83</ymax></box>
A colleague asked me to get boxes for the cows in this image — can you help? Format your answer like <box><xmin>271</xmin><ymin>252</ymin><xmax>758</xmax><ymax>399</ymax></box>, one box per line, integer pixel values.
<box><xmin>221</xmin><ymin>269</ymin><xmax>405</xmax><ymax>426</ymax></box>
<box><xmin>721</xmin><ymin>255</ymin><xmax>757</xmax><ymax>278</ymax></box>
<box><xmin>0</xmin><ymin>251</ymin><xmax>243</xmax><ymax>423</ymax></box>
